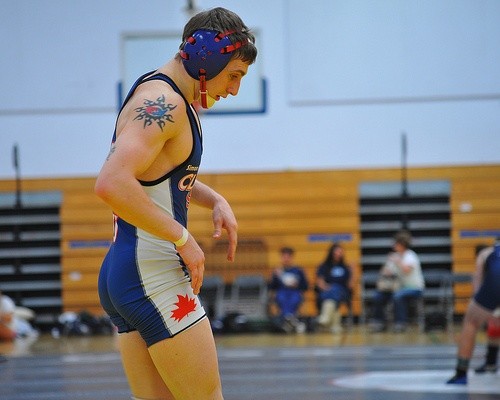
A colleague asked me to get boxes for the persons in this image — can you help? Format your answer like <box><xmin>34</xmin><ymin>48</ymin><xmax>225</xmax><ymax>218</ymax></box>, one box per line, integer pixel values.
<box><xmin>97</xmin><ymin>6</ymin><xmax>257</xmax><ymax>400</ymax></box>
<box><xmin>474</xmin><ymin>243</ymin><xmax>500</xmax><ymax>373</ymax></box>
<box><xmin>313</xmin><ymin>243</ymin><xmax>353</xmax><ymax>333</ymax></box>
<box><xmin>267</xmin><ymin>246</ymin><xmax>308</xmax><ymax>336</ymax></box>
<box><xmin>445</xmin><ymin>235</ymin><xmax>500</xmax><ymax>385</ymax></box>
<box><xmin>368</xmin><ymin>231</ymin><xmax>425</xmax><ymax>334</ymax></box>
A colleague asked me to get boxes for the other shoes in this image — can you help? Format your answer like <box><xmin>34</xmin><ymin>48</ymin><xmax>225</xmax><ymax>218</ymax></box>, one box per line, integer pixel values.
<box><xmin>447</xmin><ymin>374</ymin><xmax>466</xmax><ymax>384</ymax></box>
<box><xmin>475</xmin><ymin>362</ymin><xmax>497</xmax><ymax>373</ymax></box>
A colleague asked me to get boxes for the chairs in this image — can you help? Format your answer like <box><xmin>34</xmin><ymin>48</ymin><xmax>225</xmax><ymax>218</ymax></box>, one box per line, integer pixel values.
<box><xmin>198</xmin><ymin>272</ymin><xmax>472</xmax><ymax>336</ymax></box>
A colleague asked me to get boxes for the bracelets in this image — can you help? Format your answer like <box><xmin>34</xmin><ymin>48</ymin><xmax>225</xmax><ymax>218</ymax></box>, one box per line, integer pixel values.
<box><xmin>173</xmin><ymin>227</ymin><xmax>189</xmax><ymax>247</ymax></box>
